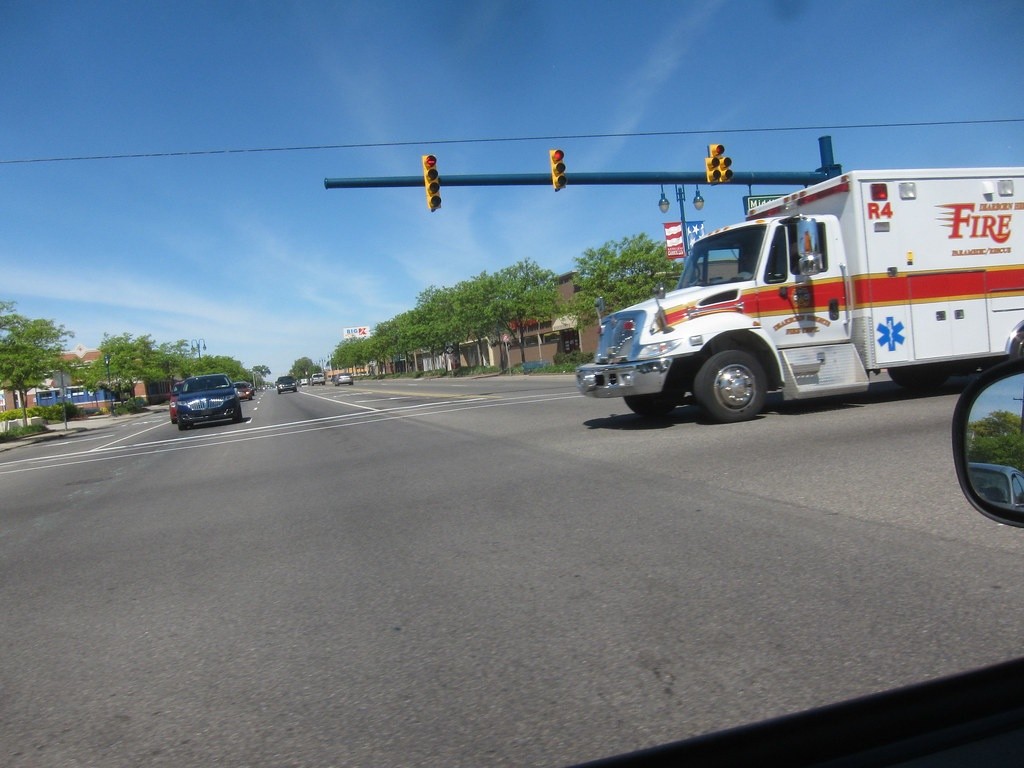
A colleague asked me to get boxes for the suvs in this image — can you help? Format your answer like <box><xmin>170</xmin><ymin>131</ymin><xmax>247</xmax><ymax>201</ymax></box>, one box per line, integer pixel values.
<box><xmin>311</xmin><ymin>372</ymin><xmax>326</xmax><ymax>386</ymax></box>
<box><xmin>275</xmin><ymin>375</ymin><xmax>298</xmax><ymax>394</ymax></box>
<box><xmin>171</xmin><ymin>373</ymin><xmax>243</xmax><ymax>431</ymax></box>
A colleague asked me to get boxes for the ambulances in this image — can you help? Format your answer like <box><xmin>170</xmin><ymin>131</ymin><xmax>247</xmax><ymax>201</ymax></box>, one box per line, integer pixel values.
<box><xmin>575</xmin><ymin>166</ymin><xmax>1024</xmax><ymax>424</ymax></box>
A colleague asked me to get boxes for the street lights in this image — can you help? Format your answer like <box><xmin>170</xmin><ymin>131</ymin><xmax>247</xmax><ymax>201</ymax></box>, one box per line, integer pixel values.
<box><xmin>191</xmin><ymin>338</ymin><xmax>207</xmax><ymax>375</ymax></box>
<box><xmin>659</xmin><ymin>184</ymin><xmax>705</xmax><ymax>255</ymax></box>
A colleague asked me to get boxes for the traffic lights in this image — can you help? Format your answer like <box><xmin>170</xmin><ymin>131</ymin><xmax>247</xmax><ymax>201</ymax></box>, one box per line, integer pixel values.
<box><xmin>719</xmin><ymin>156</ymin><xmax>734</xmax><ymax>182</ymax></box>
<box><xmin>705</xmin><ymin>157</ymin><xmax>721</xmax><ymax>183</ymax></box>
<box><xmin>423</xmin><ymin>154</ymin><xmax>442</xmax><ymax>209</ymax></box>
<box><xmin>708</xmin><ymin>144</ymin><xmax>725</xmax><ymax>158</ymax></box>
<box><xmin>550</xmin><ymin>149</ymin><xmax>568</xmax><ymax>190</ymax></box>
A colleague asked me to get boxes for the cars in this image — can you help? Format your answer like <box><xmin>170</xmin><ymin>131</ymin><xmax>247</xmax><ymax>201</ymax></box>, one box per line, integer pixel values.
<box><xmin>300</xmin><ymin>378</ymin><xmax>309</xmax><ymax>385</ymax></box>
<box><xmin>166</xmin><ymin>380</ymin><xmax>186</xmax><ymax>424</ymax></box>
<box><xmin>334</xmin><ymin>373</ymin><xmax>354</xmax><ymax>386</ymax></box>
<box><xmin>232</xmin><ymin>381</ymin><xmax>254</xmax><ymax>400</ymax></box>
<box><xmin>967</xmin><ymin>462</ymin><xmax>1023</xmax><ymax>513</ymax></box>
